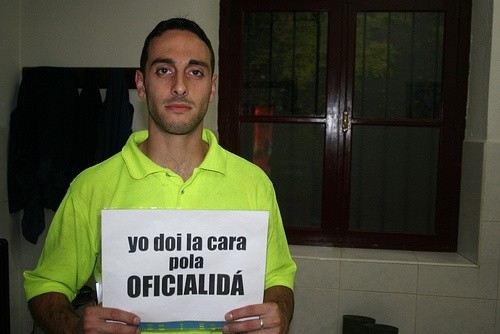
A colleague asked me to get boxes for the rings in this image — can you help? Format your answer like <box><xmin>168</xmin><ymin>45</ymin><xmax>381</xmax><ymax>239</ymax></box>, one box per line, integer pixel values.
<box><xmin>260</xmin><ymin>317</ymin><xmax>264</xmax><ymax>329</ymax></box>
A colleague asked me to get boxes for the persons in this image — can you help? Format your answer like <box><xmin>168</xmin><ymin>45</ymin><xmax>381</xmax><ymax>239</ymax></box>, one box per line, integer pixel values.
<box><xmin>22</xmin><ymin>17</ymin><xmax>297</xmax><ymax>334</ymax></box>
